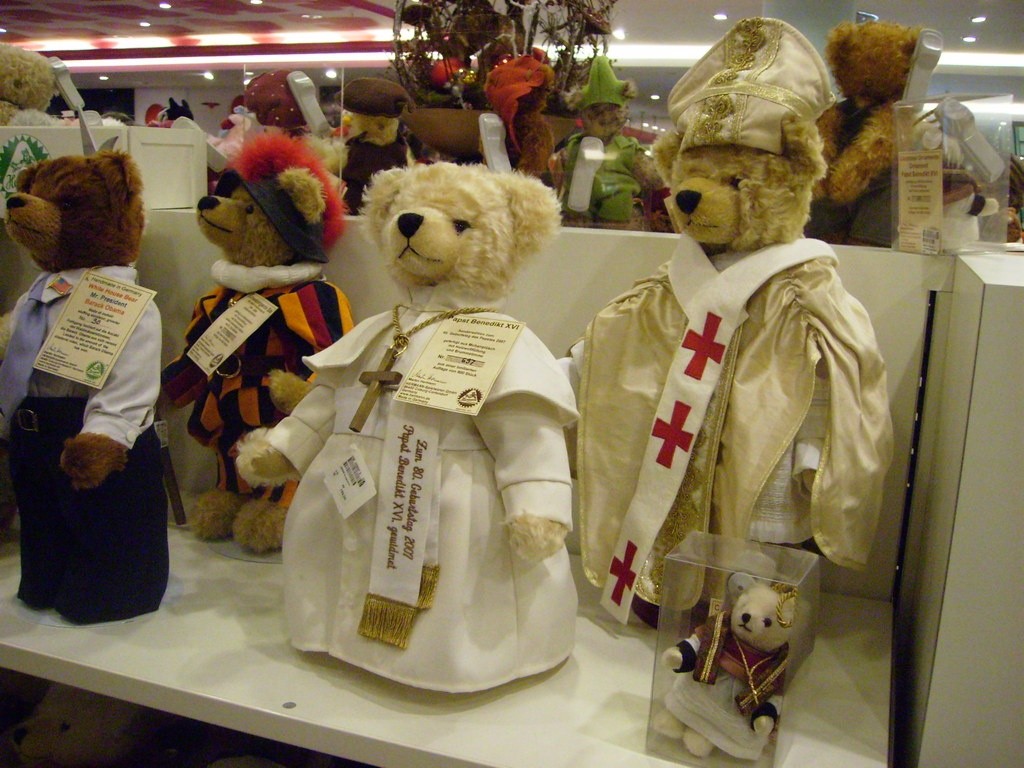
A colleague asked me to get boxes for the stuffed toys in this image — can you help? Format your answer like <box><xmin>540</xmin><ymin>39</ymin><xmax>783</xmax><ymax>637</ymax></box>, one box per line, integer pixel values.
<box><xmin>805</xmin><ymin>22</ymin><xmax>983</xmax><ymax>249</ymax></box>
<box><xmin>0</xmin><ymin>49</ymin><xmax>669</xmax><ymax>231</ymax></box>
<box><xmin>234</xmin><ymin>163</ymin><xmax>579</xmax><ymax>696</ymax></box>
<box><xmin>161</xmin><ymin>133</ymin><xmax>355</xmax><ymax>556</ymax></box>
<box><xmin>0</xmin><ymin>149</ymin><xmax>169</xmax><ymax>624</ymax></box>
<box><xmin>558</xmin><ymin>16</ymin><xmax>893</xmax><ymax>628</ymax></box>
<box><xmin>657</xmin><ymin>572</ymin><xmax>809</xmax><ymax>761</ymax></box>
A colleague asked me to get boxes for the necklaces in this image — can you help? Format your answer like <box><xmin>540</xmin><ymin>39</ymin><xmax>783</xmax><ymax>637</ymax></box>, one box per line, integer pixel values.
<box><xmin>349</xmin><ymin>303</ymin><xmax>495</xmax><ymax>433</ymax></box>
